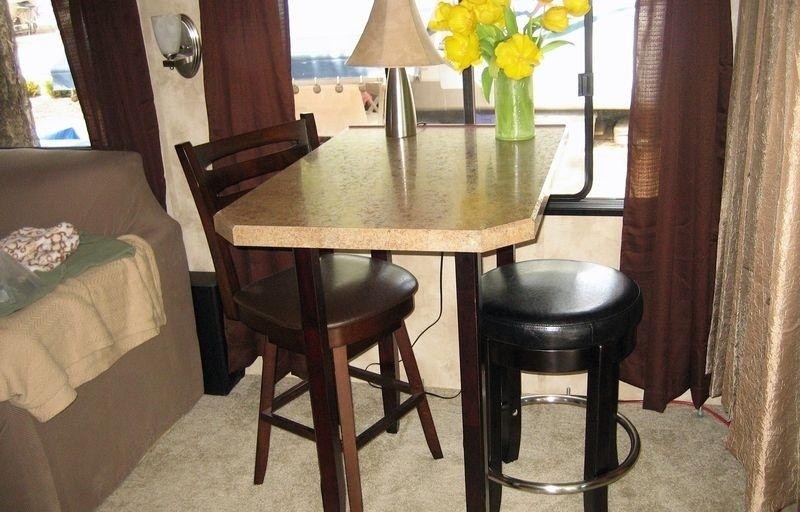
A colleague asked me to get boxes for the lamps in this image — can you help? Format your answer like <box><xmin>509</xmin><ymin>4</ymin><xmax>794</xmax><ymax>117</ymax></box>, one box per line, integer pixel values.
<box><xmin>149</xmin><ymin>12</ymin><xmax>201</xmax><ymax>79</ymax></box>
<box><xmin>343</xmin><ymin>0</ymin><xmax>445</xmax><ymax>138</ymax></box>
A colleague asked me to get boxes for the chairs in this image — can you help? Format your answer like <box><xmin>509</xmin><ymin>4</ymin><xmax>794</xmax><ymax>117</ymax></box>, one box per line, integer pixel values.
<box><xmin>173</xmin><ymin>111</ymin><xmax>443</xmax><ymax>511</ymax></box>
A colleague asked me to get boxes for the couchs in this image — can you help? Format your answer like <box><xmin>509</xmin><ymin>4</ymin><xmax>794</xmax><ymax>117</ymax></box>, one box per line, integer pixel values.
<box><xmin>0</xmin><ymin>148</ymin><xmax>206</xmax><ymax>512</ymax></box>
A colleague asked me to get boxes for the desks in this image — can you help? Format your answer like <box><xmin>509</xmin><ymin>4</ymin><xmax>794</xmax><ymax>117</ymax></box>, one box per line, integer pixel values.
<box><xmin>213</xmin><ymin>123</ymin><xmax>570</xmax><ymax>512</ymax></box>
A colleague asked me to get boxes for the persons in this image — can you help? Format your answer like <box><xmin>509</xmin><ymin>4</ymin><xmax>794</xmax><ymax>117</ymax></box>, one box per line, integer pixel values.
<box><xmin>362</xmin><ymin>88</ymin><xmax>379</xmax><ymax>113</ymax></box>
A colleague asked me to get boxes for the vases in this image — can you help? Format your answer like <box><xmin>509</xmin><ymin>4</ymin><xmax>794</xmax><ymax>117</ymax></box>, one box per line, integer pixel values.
<box><xmin>493</xmin><ymin>77</ymin><xmax>535</xmax><ymax>142</ymax></box>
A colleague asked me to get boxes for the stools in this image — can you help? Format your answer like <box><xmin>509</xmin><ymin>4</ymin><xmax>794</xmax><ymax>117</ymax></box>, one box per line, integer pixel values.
<box><xmin>478</xmin><ymin>259</ymin><xmax>643</xmax><ymax>511</ymax></box>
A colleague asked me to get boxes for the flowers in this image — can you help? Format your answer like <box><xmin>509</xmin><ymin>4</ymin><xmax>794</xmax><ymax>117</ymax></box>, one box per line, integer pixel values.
<box><xmin>427</xmin><ymin>0</ymin><xmax>591</xmax><ymax>104</ymax></box>
